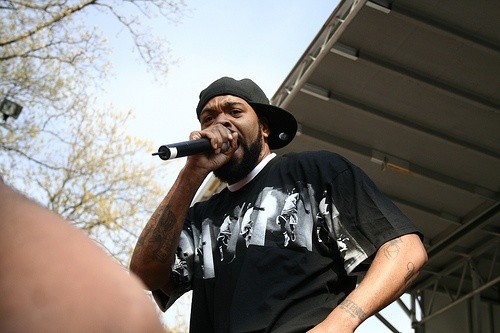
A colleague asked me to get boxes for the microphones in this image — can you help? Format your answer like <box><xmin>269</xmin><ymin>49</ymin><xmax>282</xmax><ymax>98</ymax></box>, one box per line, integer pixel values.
<box><xmin>152</xmin><ymin>136</ymin><xmax>231</xmax><ymax>161</ymax></box>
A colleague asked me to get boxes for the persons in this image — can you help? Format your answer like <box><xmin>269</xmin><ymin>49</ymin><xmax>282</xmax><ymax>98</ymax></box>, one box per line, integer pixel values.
<box><xmin>0</xmin><ymin>178</ymin><xmax>169</xmax><ymax>333</ymax></box>
<box><xmin>130</xmin><ymin>77</ymin><xmax>428</xmax><ymax>333</ymax></box>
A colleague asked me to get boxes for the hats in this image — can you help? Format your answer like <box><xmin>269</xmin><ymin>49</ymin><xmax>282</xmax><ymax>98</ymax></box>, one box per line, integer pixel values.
<box><xmin>197</xmin><ymin>77</ymin><xmax>298</xmax><ymax>150</ymax></box>
